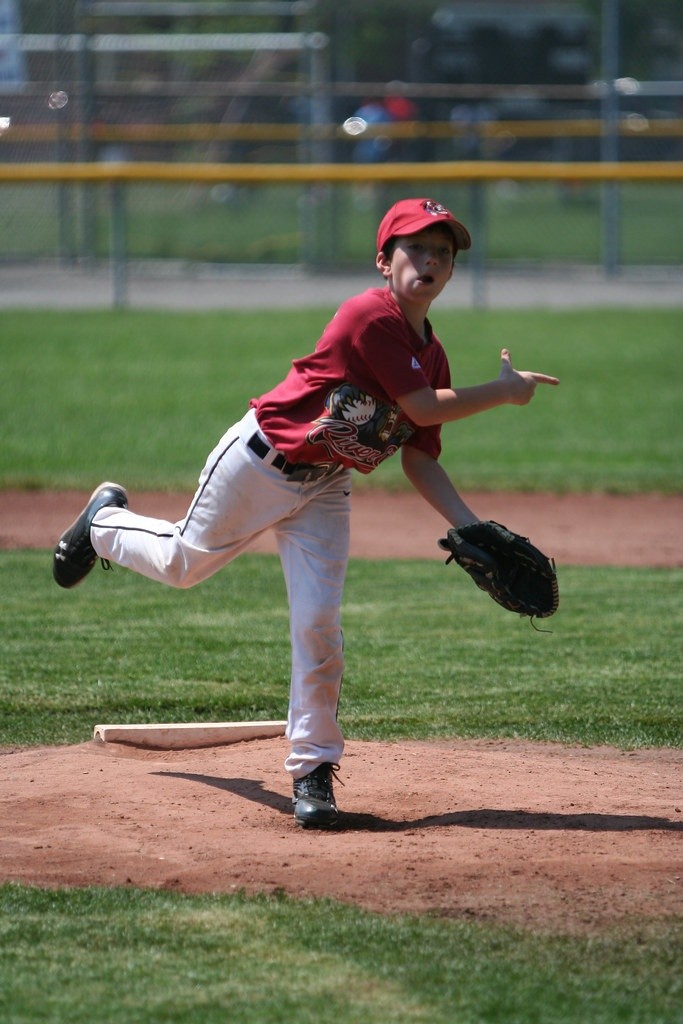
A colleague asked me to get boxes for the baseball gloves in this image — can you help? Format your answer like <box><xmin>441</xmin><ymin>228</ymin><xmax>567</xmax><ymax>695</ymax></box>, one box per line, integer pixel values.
<box><xmin>435</xmin><ymin>518</ymin><xmax>562</xmax><ymax>635</ymax></box>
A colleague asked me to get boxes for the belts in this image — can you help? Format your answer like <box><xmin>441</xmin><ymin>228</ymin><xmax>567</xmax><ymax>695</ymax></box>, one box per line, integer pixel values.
<box><xmin>246</xmin><ymin>433</ymin><xmax>339</xmax><ymax>482</ymax></box>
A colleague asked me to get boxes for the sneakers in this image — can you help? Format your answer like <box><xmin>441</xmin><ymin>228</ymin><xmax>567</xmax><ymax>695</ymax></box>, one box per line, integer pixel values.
<box><xmin>52</xmin><ymin>482</ymin><xmax>128</xmax><ymax>589</ymax></box>
<box><xmin>292</xmin><ymin>762</ymin><xmax>345</xmax><ymax>828</ymax></box>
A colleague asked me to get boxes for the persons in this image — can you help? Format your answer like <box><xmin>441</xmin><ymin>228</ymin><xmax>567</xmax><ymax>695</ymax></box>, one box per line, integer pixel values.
<box><xmin>51</xmin><ymin>199</ymin><xmax>562</xmax><ymax>830</ymax></box>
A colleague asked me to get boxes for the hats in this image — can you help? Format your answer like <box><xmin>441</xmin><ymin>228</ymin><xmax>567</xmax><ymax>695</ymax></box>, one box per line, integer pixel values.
<box><xmin>376</xmin><ymin>198</ymin><xmax>472</xmax><ymax>253</ymax></box>
